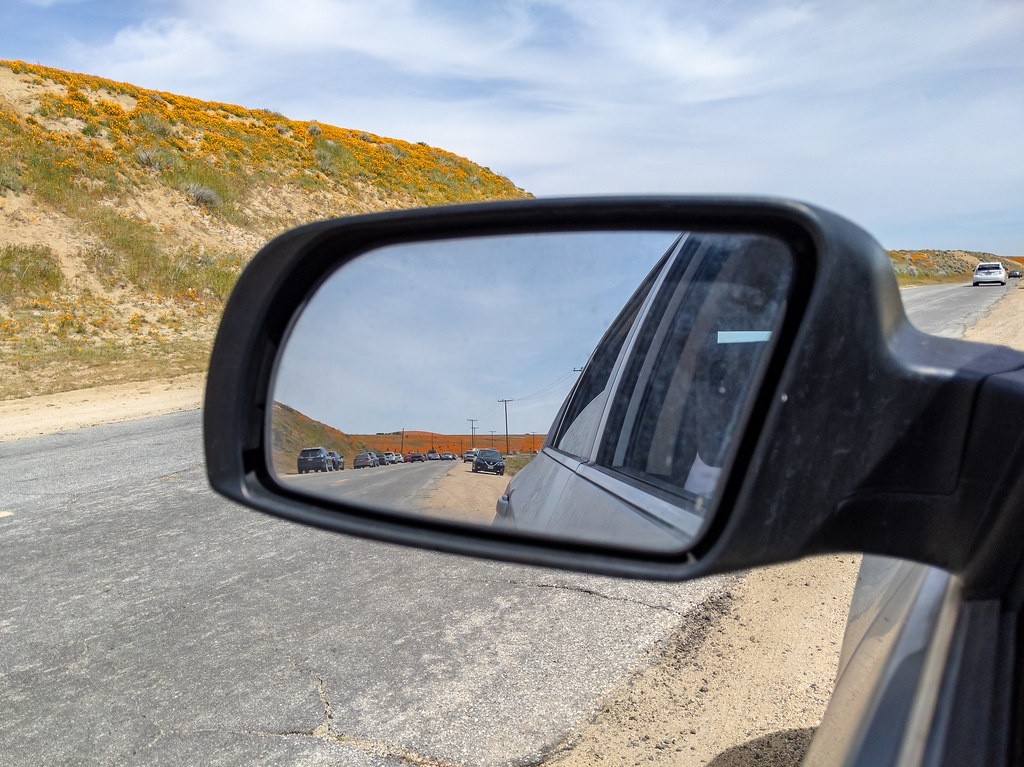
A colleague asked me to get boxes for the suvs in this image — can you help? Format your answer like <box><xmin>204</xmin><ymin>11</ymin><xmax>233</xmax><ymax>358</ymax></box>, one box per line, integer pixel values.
<box><xmin>464</xmin><ymin>451</ymin><xmax>476</xmax><ymax>463</ymax></box>
<box><xmin>362</xmin><ymin>452</ymin><xmax>380</xmax><ymax>467</ymax></box>
<box><xmin>297</xmin><ymin>447</ymin><xmax>333</xmax><ymax>473</ymax></box>
<box><xmin>383</xmin><ymin>452</ymin><xmax>397</xmax><ymax>464</ymax></box>
<box><xmin>472</xmin><ymin>450</ymin><xmax>506</xmax><ymax>475</ymax></box>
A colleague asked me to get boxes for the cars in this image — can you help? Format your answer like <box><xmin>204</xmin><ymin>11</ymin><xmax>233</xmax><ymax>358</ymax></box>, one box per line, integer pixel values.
<box><xmin>376</xmin><ymin>454</ymin><xmax>390</xmax><ymax>466</ymax></box>
<box><xmin>1009</xmin><ymin>270</ymin><xmax>1020</xmax><ymax>277</ymax></box>
<box><xmin>506</xmin><ymin>450</ymin><xmax>538</xmax><ymax>455</ymax></box>
<box><xmin>394</xmin><ymin>453</ymin><xmax>405</xmax><ymax>463</ymax></box>
<box><xmin>972</xmin><ymin>262</ymin><xmax>1007</xmax><ymax>287</ymax></box>
<box><xmin>491</xmin><ymin>232</ymin><xmax>795</xmax><ymax>552</ymax></box>
<box><xmin>404</xmin><ymin>452</ymin><xmax>458</xmax><ymax>462</ymax></box>
<box><xmin>327</xmin><ymin>451</ymin><xmax>344</xmax><ymax>470</ymax></box>
<box><xmin>411</xmin><ymin>453</ymin><xmax>425</xmax><ymax>463</ymax></box>
<box><xmin>353</xmin><ymin>454</ymin><xmax>372</xmax><ymax>469</ymax></box>
